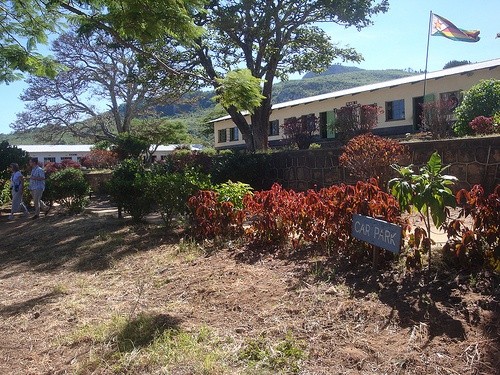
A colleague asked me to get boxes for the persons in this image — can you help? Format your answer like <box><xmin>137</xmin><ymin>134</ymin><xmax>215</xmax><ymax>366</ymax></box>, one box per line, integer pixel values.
<box><xmin>25</xmin><ymin>157</ymin><xmax>53</xmax><ymax>220</ymax></box>
<box><xmin>4</xmin><ymin>163</ymin><xmax>33</xmax><ymax>221</ymax></box>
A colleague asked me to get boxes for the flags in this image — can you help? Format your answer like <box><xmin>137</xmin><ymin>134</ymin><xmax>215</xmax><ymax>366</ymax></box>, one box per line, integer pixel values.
<box><xmin>431</xmin><ymin>12</ymin><xmax>482</xmax><ymax>44</ymax></box>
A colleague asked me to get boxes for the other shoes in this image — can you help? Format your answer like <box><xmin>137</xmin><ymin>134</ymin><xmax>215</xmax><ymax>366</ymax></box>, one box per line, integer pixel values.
<box><xmin>21</xmin><ymin>211</ymin><xmax>30</xmax><ymax>217</ymax></box>
<box><xmin>45</xmin><ymin>207</ymin><xmax>50</xmax><ymax>216</ymax></box>
<box><xmin>31</xmin><ymin>214</ymin><xmax>39</xmax><ymax>219</ymax></box>
<box><xmin>8</xmin><ymin>216</ymin><xmax>15</xmax><ymax>221</ymax></box>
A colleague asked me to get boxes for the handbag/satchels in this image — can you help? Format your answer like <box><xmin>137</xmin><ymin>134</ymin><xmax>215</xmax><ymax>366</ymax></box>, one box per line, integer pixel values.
<box><xmin>14</xmin><ymin>184</ymin><xmax>20</xmax><ymax>192</ymax></box>
